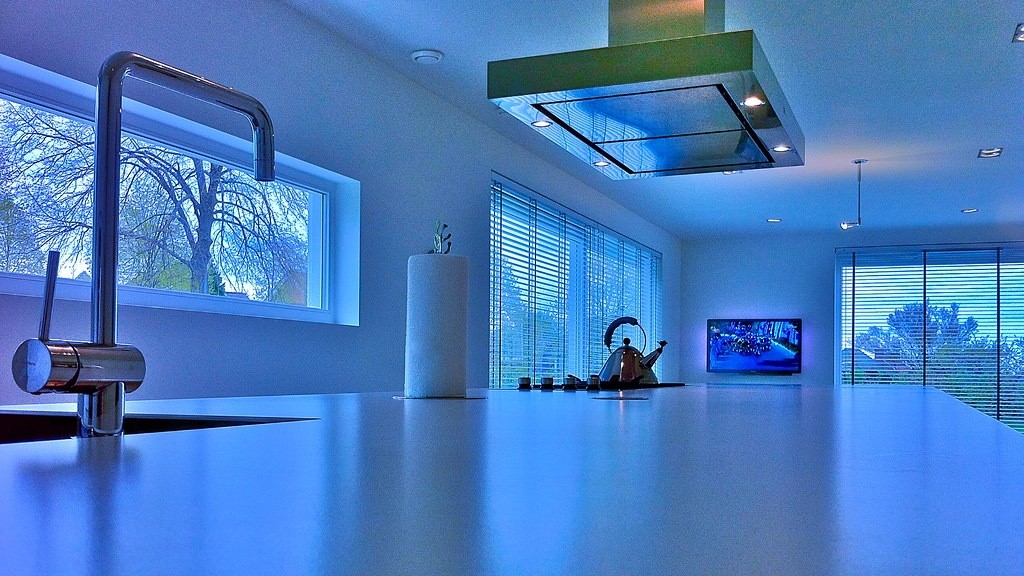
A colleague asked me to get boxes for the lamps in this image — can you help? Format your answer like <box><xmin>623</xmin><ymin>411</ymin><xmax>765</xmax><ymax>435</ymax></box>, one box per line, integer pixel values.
<box><xmin>840</xmin><ymin>159</ymin><xmax>868</xmax><ymax>230</ymax></box>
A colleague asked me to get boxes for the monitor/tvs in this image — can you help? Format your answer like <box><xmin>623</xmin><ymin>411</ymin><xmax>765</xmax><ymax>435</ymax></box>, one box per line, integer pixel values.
<box><xmin>707</xmin><ymin>319</ymin><xmax>802</xmax><ymax>376</ymax></box>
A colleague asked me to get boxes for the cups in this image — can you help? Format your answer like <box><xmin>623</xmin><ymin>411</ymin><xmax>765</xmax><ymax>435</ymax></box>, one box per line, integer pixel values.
<box><xmin>586</xmin><ymin>378</ymin><xmax>602</xmax><ymax>391</ymax></box>
<box><xmin>562</xmin><ymin>377</ymin><xmax>578</xmax><ymax>391</ymax></box>
<box><xmin>517</xmin><ymin>377</ymin><xmax>532</xmax><ymax>391</ymax></box>
<box><xmin>540</xmin><ymin>377</ymin><xmax>554</xmax><ymax>391</ymax></box>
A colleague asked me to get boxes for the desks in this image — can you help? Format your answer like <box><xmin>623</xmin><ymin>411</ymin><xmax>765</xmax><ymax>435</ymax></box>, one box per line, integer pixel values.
<box><xmin>0</xmin><ymin>383</ymin><xmax>1024</xmax><ymax>576</ymax></box>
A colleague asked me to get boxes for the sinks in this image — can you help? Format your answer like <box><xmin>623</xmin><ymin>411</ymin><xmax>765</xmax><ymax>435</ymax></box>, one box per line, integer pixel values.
<box><xmin>0</xmin><ymin>410</ymin><xmax>321</xmax><ymax>445</ymax></box>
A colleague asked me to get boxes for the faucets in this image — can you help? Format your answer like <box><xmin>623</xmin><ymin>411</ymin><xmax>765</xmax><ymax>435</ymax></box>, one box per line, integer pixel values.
<box><xmin>10</xmin><ymin>50</ymin><xmax>277</xmax><ymax>438</ymax></box>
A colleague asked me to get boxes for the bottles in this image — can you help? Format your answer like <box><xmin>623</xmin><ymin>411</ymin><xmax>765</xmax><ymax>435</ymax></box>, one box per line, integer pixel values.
<box><xmin>620</xmin><ymin>350</ymin><xmax>637</xmax><ymax>383</ymax></box>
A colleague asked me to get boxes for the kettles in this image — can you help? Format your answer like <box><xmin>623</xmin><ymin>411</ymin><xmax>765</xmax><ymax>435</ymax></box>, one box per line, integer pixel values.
<box><xmin>596</xmin><ymin>316</ymin><xmax>668</xmax><ymax>386</ymax></box>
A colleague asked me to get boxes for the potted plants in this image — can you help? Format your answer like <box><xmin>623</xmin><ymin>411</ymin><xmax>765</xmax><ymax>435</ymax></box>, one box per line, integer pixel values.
<box><xmin>404</xmin><ymin>219</ymin><xmax>468</xmax><ymax>398</ymax></box>
